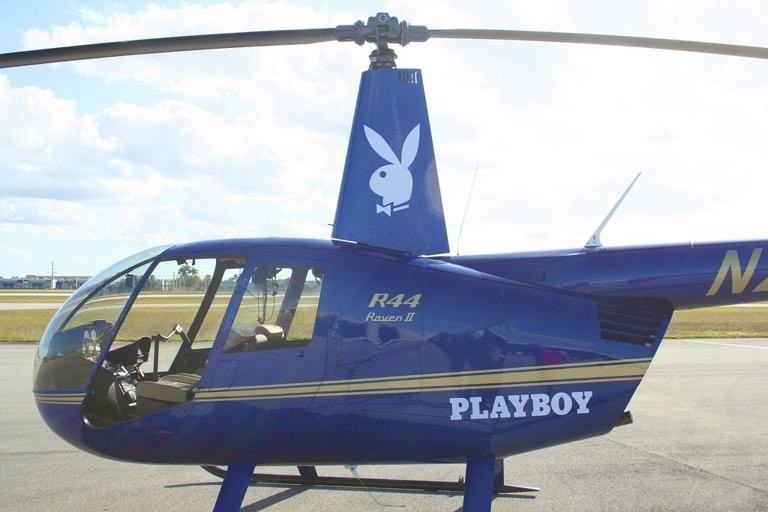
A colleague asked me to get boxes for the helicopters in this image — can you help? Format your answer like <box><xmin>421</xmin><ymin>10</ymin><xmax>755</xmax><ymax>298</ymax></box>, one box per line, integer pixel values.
<box><xmin>0</xmin><ymin>12</ymin><xmax>768</xmax><ymax>512</ymax></box>
